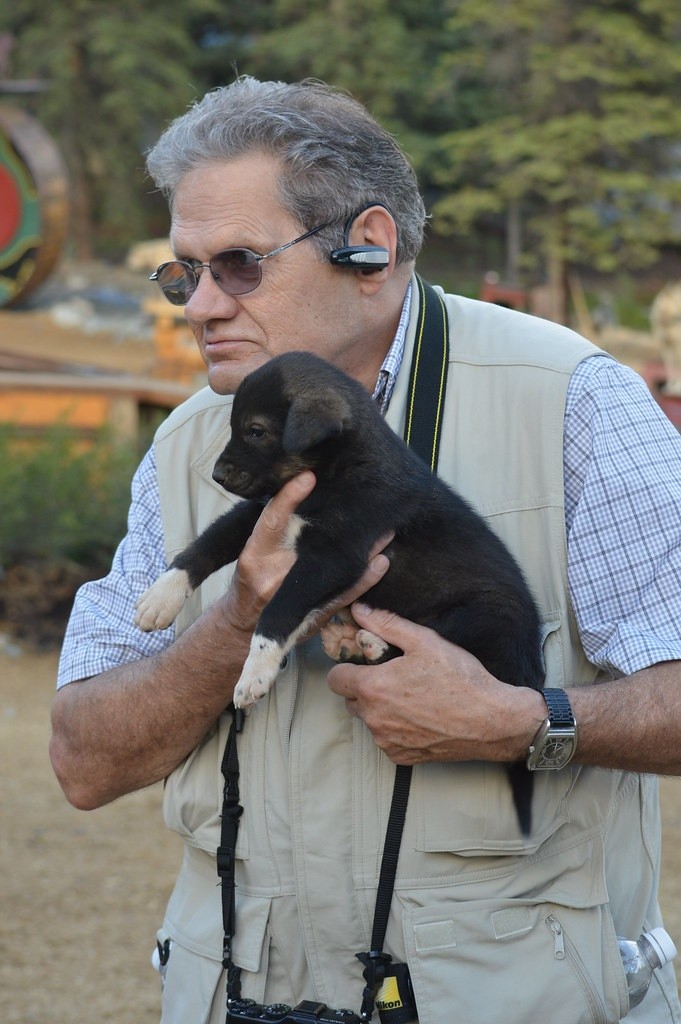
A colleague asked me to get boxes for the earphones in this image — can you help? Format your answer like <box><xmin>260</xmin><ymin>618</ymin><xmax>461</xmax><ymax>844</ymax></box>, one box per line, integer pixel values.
<box><xmin>330</xmin><ymin>245</ymin><xmax>390</xmax><ymax>270</ymax></box>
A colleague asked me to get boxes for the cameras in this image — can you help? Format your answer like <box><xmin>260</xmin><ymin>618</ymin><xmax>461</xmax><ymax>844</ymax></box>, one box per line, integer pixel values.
<box><xmin>225</xmin><ymin>997</ymin><xmax>360</xmax><ymax>1024</ymax></box>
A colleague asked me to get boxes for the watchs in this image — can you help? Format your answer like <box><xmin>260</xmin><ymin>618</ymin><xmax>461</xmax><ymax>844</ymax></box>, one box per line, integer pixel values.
<box><xmin>523</xmin><ymin>687</ymin><xmax>579</xmax><ymax>772</ymax></box>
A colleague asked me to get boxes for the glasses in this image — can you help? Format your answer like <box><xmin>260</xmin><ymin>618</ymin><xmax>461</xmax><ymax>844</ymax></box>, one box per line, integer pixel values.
<box><xmin>150</xmin><ymin>211</ymin><xmax>352</xmax><ymax>305</ymax></box>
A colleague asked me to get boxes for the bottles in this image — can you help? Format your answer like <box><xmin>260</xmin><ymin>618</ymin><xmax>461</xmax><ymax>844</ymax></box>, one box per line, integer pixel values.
<box><xmin>616</xmin><ymin>926</ymin><xmax>678</xmax><ymax>1014</ymax></box>
<box><xmin>151</xmin><ymin>943</ymin><xmax>168</xmax><ymax>986</ymax></box>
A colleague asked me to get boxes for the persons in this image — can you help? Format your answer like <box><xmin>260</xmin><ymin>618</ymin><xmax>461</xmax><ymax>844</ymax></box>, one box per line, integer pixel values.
<box><xmin>639</xmin><ymin>277</ymin><xmax>681</xmax><ymax>434</ymax></box>
<box><xmin>48</xmin><ymin>75</ymin><xmax>680</xmax><ymax>1024</ymax></box>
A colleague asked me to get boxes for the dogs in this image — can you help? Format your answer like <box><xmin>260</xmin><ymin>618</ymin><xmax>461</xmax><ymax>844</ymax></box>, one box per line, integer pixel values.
<box><xmin>130</xmin><ymin>352</ymin><xmax>543</xmax><ymax>837</ymax></box>
